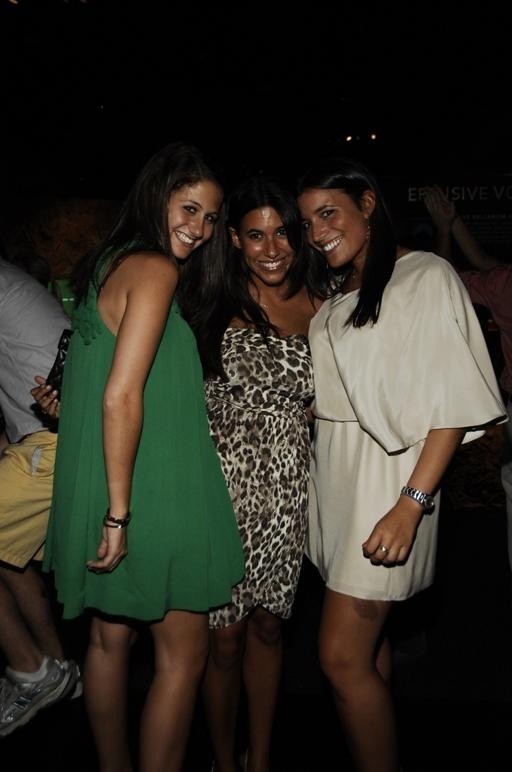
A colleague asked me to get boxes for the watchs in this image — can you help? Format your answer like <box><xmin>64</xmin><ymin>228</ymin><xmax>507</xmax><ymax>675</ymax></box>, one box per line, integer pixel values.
<box><xmin>401</xmin><ymin>487</ymin><xmax>435</xmax><ymax>515</ymax></box>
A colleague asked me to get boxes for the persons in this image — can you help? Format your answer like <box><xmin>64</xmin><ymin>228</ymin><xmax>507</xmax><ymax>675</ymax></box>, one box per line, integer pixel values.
<box><xmin>295</xmin><ymin>154</ymin><xmax>509</xmax><ymax>771</ymax></box>
<box><xmin>41</xmin><ymin>141</ymin><xmax>247</xmax><ymax>772</ymax></box>
<box><xmin>0</xmin><ymin>256</ymin><xmax>83</xmax><ymax>743</ymax></box>
<box><xmin>31</xmin><ymin>175</ymin><xmax>326</xmax><ymax>772</ymax></box>
<box><xmin>417</xmin><ymin>184</ymin><xmax>510</xmax><ymax>411</ymax></box>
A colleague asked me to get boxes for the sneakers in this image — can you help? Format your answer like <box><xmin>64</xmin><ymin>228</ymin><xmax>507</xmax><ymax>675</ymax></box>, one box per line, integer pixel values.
<box><xmin>0</xmin><ymin>657</ymin><xmax>83</xmax><ymax>737</ymax></box>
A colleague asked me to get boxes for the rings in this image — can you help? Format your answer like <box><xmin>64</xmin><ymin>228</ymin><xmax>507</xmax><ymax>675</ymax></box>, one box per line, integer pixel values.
<box><xmin>379</xmin><ymin>546</ymin><xmax>388</xmax><ymax>553</ymax></box>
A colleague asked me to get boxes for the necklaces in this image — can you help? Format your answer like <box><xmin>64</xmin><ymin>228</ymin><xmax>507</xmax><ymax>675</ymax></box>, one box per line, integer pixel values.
<box><xmin>349</xmin><ymin>272</ymin><xmax>362</xmax><ymax>281</ymax></box>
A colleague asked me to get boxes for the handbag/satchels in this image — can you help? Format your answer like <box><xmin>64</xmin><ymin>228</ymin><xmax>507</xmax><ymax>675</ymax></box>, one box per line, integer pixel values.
<box><xmin>46</xmin><ymin>329</ymin><xmax>74</xmax><ymax>401</ymax></box>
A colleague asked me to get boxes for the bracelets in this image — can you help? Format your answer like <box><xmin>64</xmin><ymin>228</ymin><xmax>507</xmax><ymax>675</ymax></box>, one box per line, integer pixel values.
<box><xmin>103</xmin><ymin>508</ymin><xmax>131</xmax><ymax>528</ymax></box>
